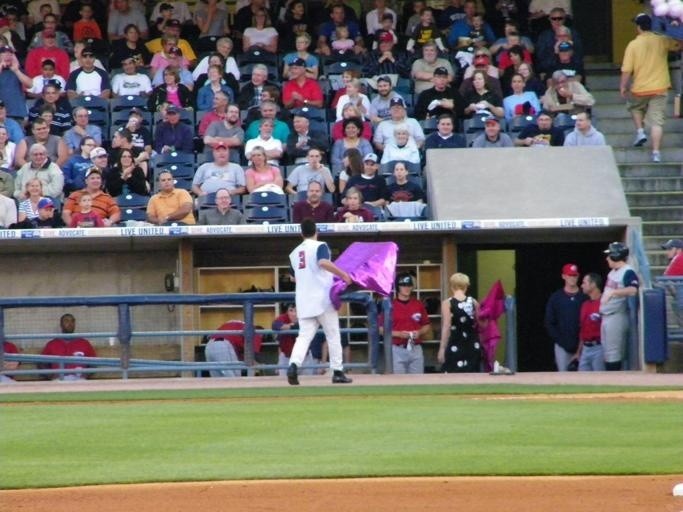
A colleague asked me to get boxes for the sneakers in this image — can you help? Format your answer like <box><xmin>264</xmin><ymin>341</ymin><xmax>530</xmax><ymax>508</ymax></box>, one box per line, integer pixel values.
<box><xmin>332</xmin><ymin>373</ymin><xmax>353</xmax><ymax>384</ymax></box>
<box><xmin>633</xmin><ymin>133</ymin><xmax>648</xmax><ymax>147</ymax></box>
<box><xmin>651</xmin><ymin>151</ymin><xmax>661</xmax><ymax>163</ymax></box>
<box><xmin>286</xmin><ymin>363</ymin><xmax>299</xmax><ymax>385</ymax></box>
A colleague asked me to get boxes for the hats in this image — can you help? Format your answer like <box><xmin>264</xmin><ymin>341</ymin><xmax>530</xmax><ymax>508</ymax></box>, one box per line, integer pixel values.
<box><xmin>85</xmin><ymin>166</ymin><xmax>102</xmax><ymax>178</ymax></box>
<box><xmin>397</xmin><ymin>274</ymin><xmax>413</xmax><ymax>287</ymax></box>
<box><xmin>362</xmin><ymin>152</ymin><xmax>381</xmax><ymax>165</ymax></box>
<box><xmin>630</xmin><ymin>13</ymin><xmax>652</xmax><ymax>24</ymax></box>
<box><xmin>89</xmin><ymin>146</ymin><xmax>110</xmax><ymax>158</ymax></box>
<box><xmin>166</xmin><ymin>104</ymin><xmax>180</xmax><ymax>114</ymax></box>
<box><xmin>37</xmin><ymin>198</ymin><xmax>56</xmax><ymax>209</ymax></box>
<box><xmin>213</xmin><ymin>141</ymin><xmax>228</xmax><ymax>150</ymax></box>
<box><xmin>562</xmin><ymin>263</ymin><xmax>580</xmax><ymax>276</ymax></box>
<box><xmin>603</xmin><ymin>241</ymin><xmax>629</xmax><ymax>257</ymax></box>
<box><xmin>389</xmin><ymin>97</ymin><xmax>403</xmax><ymax>107</ymax></box>
<box><xmin>129</xmin><ymin>107</ymin><xmax>143</xmax><ymax>118</ymax></box>
<box><xmin>661</xmin><ymin>239</ymin><xmax>683</xmax><ymax>250</ymax></box>
<box><xmin>485</xmin><ymin>116</ymin><xmax>499</xmax><ymax>124</ymax></box>
<box><xmin>0</xmin><ymin>0</ymin><xmax>573</xmax><ymax>94</ymax></box>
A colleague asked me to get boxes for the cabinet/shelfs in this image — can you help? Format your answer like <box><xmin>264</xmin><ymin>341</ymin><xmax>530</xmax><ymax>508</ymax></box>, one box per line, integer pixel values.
<box><xmin>192</xmin><ymin>265</ymin><xmax>446</xmax><ymax>376</ymax></box>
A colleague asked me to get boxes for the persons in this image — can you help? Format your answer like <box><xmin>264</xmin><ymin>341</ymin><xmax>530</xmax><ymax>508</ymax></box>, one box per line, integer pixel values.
<box><xmin>34</xmin><ymin>312</ymin><xmax>98</xmax><ymax>383</ymax></box>
<box><xmin>201</xmin><ymin>317</ymin><xmax>266</xmax><ymax>378</ymax></box>
<box><xmin>0</xmin><ymin>340</ymin><xmax>20</xmax><ymax>383</ymax></box>
<box><xmin>542</xmin><ymin>262</ymin><xmax>591</xmax><ymax>373</ymax></box>
<box><xmin>269</xmin><ymin>301</ymin><xmax>313</xmax><ymax>376</ymax></box>
<box><xmin>283</xmin><ymin>215</ymin><xmax>357</xmax><ymax>384</ymax></box>
<box><xmin>436</xmin><ymin>269</ymin><xmax>488</xmax><ymax>373</ymax></box>
<box><xmin>594</xmin><ymin>241</ymin><xmax>640</xmax><ymax>371</ymax></box>
<box><xmin>307</xmin><ymin>324</ymin><xmax>329</xmax><ymax>374</ymax></box>
<box><xmin>567</xmin><ymin>272</ymin><xmax>606</xmax><ymax>372</ymax></box>
<box><xmin>339</xmin><ymin>330</ymin><xmax>352</xmax><ymax>370</ymax></box>
<box><xmin>617</xmin><ymin>13</ymin><xmax>683</xmax><ymax>163</ymax></box>
<box><xmin>660</xmin><ymin>238</ymin><xmax>682</xmax><ymax>276</ymax></box>
<box><xmin>648</xmin><ymin>0</ymin><xmax>683</xmax><ymax>120</ymax></box>
<box><xmin>373</xmin><ymin>273</ymin><xmax>432</xmax><ymax>376</ymax></box>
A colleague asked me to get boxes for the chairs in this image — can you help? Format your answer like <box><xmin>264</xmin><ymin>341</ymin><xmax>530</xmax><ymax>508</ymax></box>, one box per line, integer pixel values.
<box><xmin>0</xmin><ymin>1</ymin><xmax>597</xmax><ymax>223</ymax></box>
